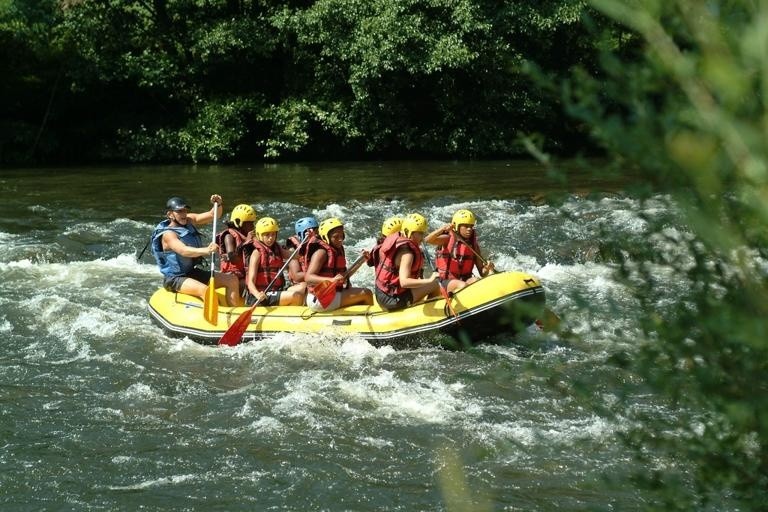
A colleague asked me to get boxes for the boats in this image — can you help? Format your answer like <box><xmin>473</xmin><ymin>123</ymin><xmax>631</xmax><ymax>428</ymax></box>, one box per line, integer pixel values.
<box><xmin>144</xmin><ymin>263</ymin><xmax>548</xmax><ymax>350</ymax></box>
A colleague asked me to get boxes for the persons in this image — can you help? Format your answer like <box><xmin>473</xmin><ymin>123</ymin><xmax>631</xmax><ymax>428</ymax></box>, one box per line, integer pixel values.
<box><xmin>241</xmin><ymin>216</ymin><xmax>304</xmax><ymax>306</ymax></box>
<box><xmin>373</xmin><ymin>212</ymin><xmax>441</xmax><ymax>311</ymax></box>
<box><xmin>360</xmin><ymin>216</ymin><xmax>402</xmax><ymax>268</ymax></box>
<box><xmin>304</xmin><ymin>216</ymin><xmax>373</xmax><ymax>313</ymax></box>
<box><xmin>285</xmin><ymin>216</ymin><xmax>319</xmax><ymax>293</ymax></box>
<box><xmin>150</xmin><ymin>193</ymin><xmax>239</xmax><ymax>307</ymax></box>
<box><xmin>215</xmin><ymin>203</ymin><xmax>257</xmax><ymax>306</ymax></box>
<box><xmin>425</xmin><ymin>208</ymin><xmax>496</xmax><ymax>293</ymax></box>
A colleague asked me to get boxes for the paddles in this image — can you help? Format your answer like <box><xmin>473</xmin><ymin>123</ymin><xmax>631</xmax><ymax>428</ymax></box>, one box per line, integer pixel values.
<box><xmin>421</xmin><ymin>240</ymin><xmax>460</xmax><ymax>325</ymax></box>
<box><xmin>217</xmin><ymin>230</ymin><xmax>313</xmax><ymax>347</ymax></box>
<box><xmin>313</xmin><ymin>249</ymin><xmax>371</xmax><ymax>309</ymax></box>
<box><xmin>450</xmin><ymin>223</ymin><xmax>560</xmax><ymax>331</ymax></box>
<box><xmin>203</xmin><ymin>196</ymin><xmax>223</xmax><ymax>326</ymax></box>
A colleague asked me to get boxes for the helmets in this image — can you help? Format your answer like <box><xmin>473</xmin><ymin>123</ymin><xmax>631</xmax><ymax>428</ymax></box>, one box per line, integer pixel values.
<box><xmin>382</xmin><ymin>217</ymin><xmax>403</xmax><ymax>238</ymax></box>
<box><xmin>294</xmin><ymin>217</ymin><xmax>319</xmax><ymax>241</ymax></box>
<box><xmin>167</xmin><ymin>197</ymin><xmax>191</xmax><ymax>213</ymax></box>
<box><xmin>401</xmin><ymin>212</ymin><xmax>427</xmax><ymax>238</ymax></box>
<box><xmin>255</xmin><ymin>217</ymin><xmax>279</xmax><ymax>241</ymax></box>
<box><xmin>319</xmin><ymin>218</ymin><xmax>345</xmax><ymax>245</ymax></box>
<box><xmin>231</xmin><ymin>204</ymin><xmax>256</xmax><ymax>227</ymax></box>
<box><xmin>452</xmin><ymin>210</ymin><xmax>475</xmax><ymax>232</ymax></box>
